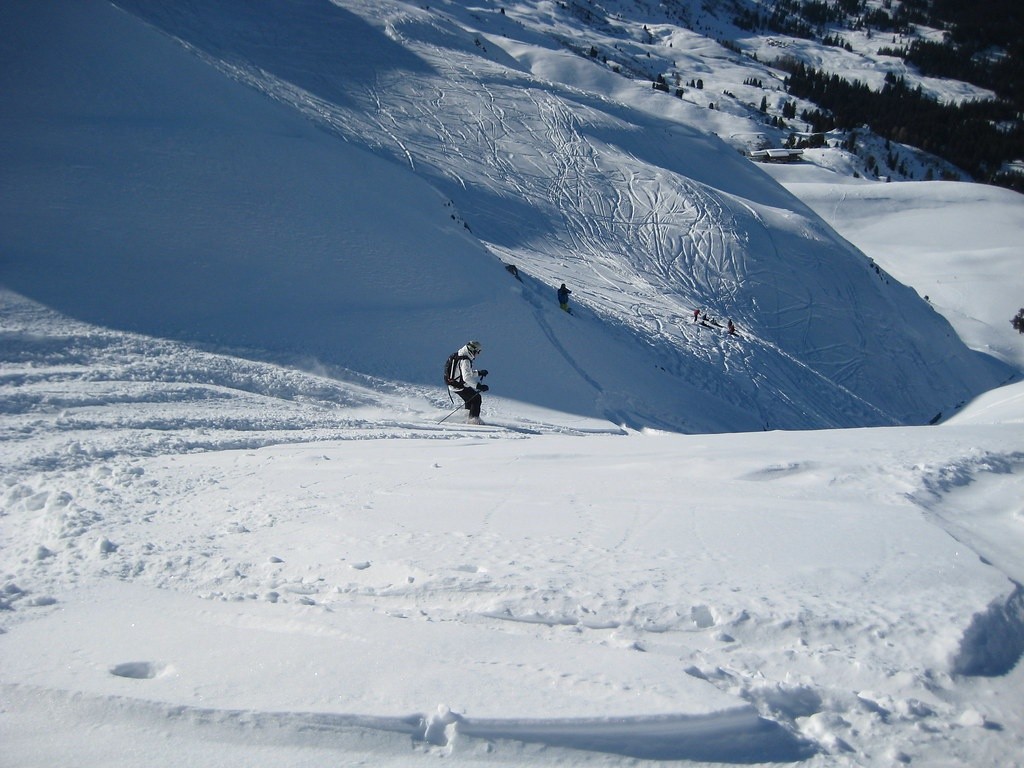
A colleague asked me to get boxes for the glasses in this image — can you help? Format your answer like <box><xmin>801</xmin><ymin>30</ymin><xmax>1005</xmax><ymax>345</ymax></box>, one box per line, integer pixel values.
<box><xmin>477</xmin><ymin>350</ymin><xmax>482</xmax><ymax>354</ymax></box>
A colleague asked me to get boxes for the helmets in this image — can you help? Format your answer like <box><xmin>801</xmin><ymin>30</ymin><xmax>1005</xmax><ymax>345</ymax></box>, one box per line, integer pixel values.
<box><xmin>467</xmin><ymin>341</ymin><xmax>481</xmax><ymax>355</ymax></box>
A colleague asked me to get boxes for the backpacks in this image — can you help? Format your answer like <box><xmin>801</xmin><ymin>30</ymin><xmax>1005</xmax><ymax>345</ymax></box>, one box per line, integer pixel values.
<box><xmin>444</xmin><ymin>352</ymin><xmax>473</xmax><ymax>389</ymax></box>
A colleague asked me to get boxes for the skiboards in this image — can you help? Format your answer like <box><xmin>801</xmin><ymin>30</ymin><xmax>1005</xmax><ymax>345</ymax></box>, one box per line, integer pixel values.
<box><xmin>465</xmin><ymin>416</ymin><xmax>487</xmax><ymax>425</ymax></box>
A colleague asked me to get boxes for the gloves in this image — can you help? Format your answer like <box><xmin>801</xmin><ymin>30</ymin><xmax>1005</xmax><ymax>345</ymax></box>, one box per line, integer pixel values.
<box><xmin>478</xmin><ymin>370</ymin><xmax>488</xmax><ymax>377</ymax></box>
<box><xmin>477</xmin><ymin>382</ymin><xmax>489</xmax><ymax>392</ymax></box>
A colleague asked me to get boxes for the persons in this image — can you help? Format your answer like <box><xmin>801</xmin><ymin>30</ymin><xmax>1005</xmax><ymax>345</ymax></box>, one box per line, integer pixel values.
<box><xmin>699</xmin><ymin>313</ymin><xmax>725</xmax><ymax>330</ymax></box>
<box><xmin>557</xmin><ymin>284</ymin><xmax>572</xmax><ymax>312</ymax></box>
<box><xmin>728</xmin><ymin>319</ymin><xmax>735</xmax><ymax>334</ymax></box>
<box><xmin>694</xmin><ymin>309</ymin><xmax>700</xmax><ymax>322</ymax></box>
<box><xmin>448</xmin><ymin>341</ymin><xmax>489</xmax><ymax>425</ymax></box>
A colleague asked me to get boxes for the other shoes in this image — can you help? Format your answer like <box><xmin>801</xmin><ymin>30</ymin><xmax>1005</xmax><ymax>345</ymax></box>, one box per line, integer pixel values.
<box><xmin>467</xmin><ymin>416</ymin><xmax>485</xmax><ymax>425</ymax></box>
